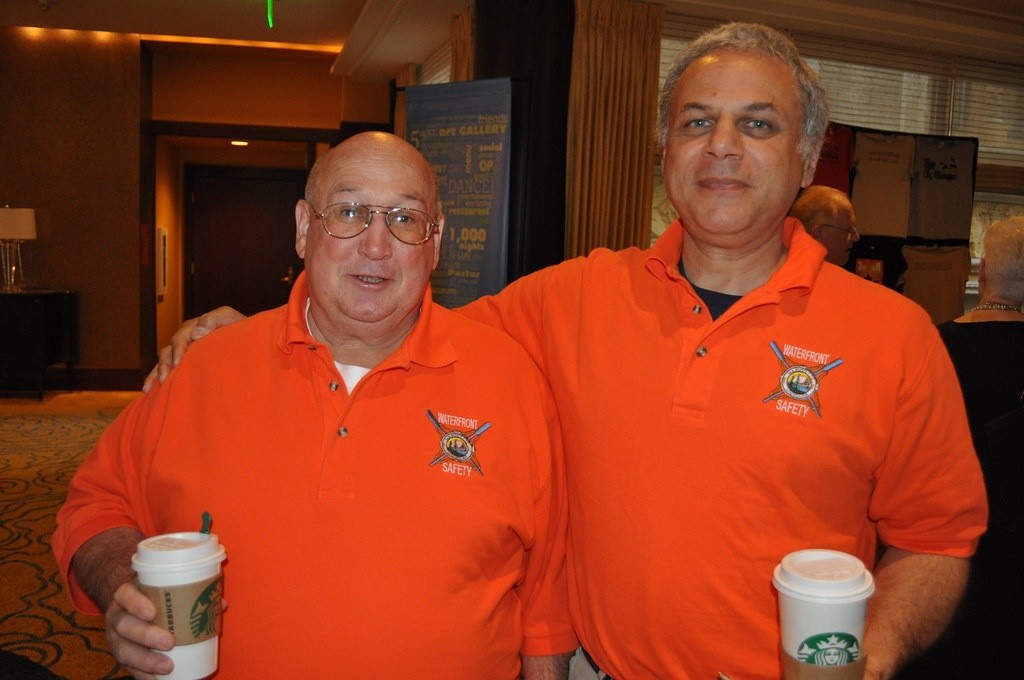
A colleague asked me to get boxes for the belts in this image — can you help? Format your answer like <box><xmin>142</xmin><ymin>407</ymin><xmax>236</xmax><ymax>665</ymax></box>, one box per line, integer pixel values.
<box><xmin>581</xmin><ymin>645</ymin><xmax>613</xmax><ymax>680</ymax></box>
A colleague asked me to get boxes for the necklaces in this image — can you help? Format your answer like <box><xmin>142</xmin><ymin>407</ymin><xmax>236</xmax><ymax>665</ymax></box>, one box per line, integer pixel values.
<box><xmin>970</xmin><ymin>302</ymin><xmax>1021</xmax><ymax>311</ymax></box>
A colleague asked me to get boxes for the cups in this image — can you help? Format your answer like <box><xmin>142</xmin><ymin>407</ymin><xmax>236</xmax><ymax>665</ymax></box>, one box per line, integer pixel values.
<box><xmin>770</xmin><ymin>549</ymin><xmax>877</xmax><ymax>680</ymax></box>
<box><xmin>130</xmin><ymin>531</ymin><xmax>227</xmax><ymax>680</ymax></box>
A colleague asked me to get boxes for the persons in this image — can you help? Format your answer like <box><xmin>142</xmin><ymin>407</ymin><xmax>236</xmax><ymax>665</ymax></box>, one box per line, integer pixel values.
<box><xmin>936</xmin><ymin>216</ymin><xmax>1024</xmax><ymax>680</ymax></box>
<box><xmin>790</xmin><ymin>185</ymin><xmax>860</xmax><ymax>265</ymax></box>
<box><xmin>50</xmin><ymin>131</ymin><xmax>579</xmax><ymax>680</ymax></box>
<box><xmin>142</xmin><ymin>23</ymin><xmax>989</xmax><ymax>680</ymax></box>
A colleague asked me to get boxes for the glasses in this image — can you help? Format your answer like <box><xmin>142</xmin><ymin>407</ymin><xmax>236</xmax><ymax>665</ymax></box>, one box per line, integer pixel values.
<box><xmin>306</xmin><ymin>200</ymin><xmax>440</xmax><ymax>245</ymax></box>
<box><xmin>819</xmin><ymin>223</ymin><xmax>859</xmax><ymax>233</ymax></box>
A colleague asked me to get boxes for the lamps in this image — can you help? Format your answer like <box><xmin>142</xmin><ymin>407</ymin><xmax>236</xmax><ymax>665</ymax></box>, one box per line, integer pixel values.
<box><xmin>0</xmin><ymin>204</ymin><xmax>38</xmax><ymax>294</ymax></box>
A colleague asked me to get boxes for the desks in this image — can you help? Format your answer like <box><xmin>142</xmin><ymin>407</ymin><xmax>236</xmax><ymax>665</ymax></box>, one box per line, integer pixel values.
<box><xmin>0</xmin><ymin>292</ymin><xmax>82</xmax><ymax>400</ymax></box>
